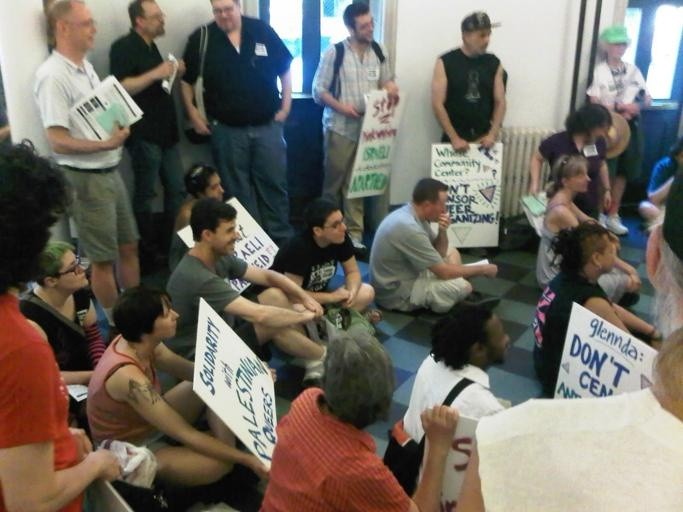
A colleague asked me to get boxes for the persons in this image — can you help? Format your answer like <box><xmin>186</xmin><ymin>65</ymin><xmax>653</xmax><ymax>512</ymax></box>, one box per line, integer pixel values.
<box><xmin>429</xmin><ymin>11</ymin><xmax>507</xmax><ymax>259</ymax></box>
<box><xmin>254</xmin><ymin>325</ymin><xmax>456</xmax><ymax>512</ymax></box>
<box><xmin>106</xmin><ymin>0</ymin><xmax>185</xmax><ymax>270</ymax></box>
<box><xmin>526</xmin><ymin>104</ymin><xmax>614</xmax><ymax>219</ymax></box>
<box><xmin>170</xmin><ymin>162</ymin><xmax>225</xmax><ymax>271</ymax></box>
<box><xmin>19</xmin><ymin>237</ymin><xmax>107</xmax><ymax>429</ymax></box>
<box><xmin>451</xmin><ymin>327</ymin><xmax>682</xmax><ymax>512</ymax></box>
<box><xmin>402</xmin><ymin>299</ymin><xmax>511</xmax><ymax>511</ymax></box>
<box><xmin>0</xmin><ymin>115</ymin><xmax>11</xmax><ymax>148</ymax></box>
<box><xmin>532</xmin><ymin>224</ymin><xmax>662</xmax><ymax>394</ymax></box>
<box><xmin>311</xmin><ymin>0</ymin><xmax>400</xmax><ymax>257</ymax></box>
<box><xmin>535</xmin><ymin>154</ymin><xmax>641</xmax><ymax>301</ymax></box>
<box><xmin>164</xmin><ymin>198</ymin><xmax>331</xmax><ymax>382</ymax></box>
<box><xmin>272</xmin><ymin>196</ymin><xmax>383</xmax><ymax>325</ymax></box>
<box><xmin>177</xmin><ymin>0</ymin><xmax>293</xmax><ymax>264</ymax></box>
<box><xmin>368</xmin><ymin>178</ymin><xmax>498</xmax><ymax>314</ymax></box>
<box><xmin>85</xmin><ymin>284</ymin><xmax>274</xmax><ymax>489</ymax></box>
<box><xmin>638</xmin><ymin>136</ymin><xmax>682</xmax><ymax>232</ymax></box>
<box><xmin>33</xmin><ymin>0</ymin><xmax>140</xmax><ymax>337</ymax></box>
<box><xmin>0</xmin><ymin>138</ymin><xmax>126</xmax><ymax>512</ymax></box>
<box><xmin>645</xmin><ymin>162</ymin><xmax>683</xmax><ymax>338</ymax></box>
<box><xmin>586</xmin><ymin>25</ymin><xmax>652</xmax><ymax>238</ymax></box>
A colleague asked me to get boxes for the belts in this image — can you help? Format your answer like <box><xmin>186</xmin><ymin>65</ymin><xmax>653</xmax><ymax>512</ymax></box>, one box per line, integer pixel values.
<box><xmin>56</xmin><ymin>163</ymin><xmax>120</xmax><ymax>174</ymax></box>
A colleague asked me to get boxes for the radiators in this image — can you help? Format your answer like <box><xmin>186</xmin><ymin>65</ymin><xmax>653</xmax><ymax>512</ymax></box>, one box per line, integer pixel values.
<box><xmin>494</xmin><ymin>127</ymin><xmax>568</xmax><ymax>218</ymax></box>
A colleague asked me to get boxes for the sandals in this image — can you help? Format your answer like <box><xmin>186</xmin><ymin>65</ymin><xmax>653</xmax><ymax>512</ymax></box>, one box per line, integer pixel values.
<box><xmin>367</xmin><ymin>307</ymin><xmax>385</xmax><ymax>323</ymax></box>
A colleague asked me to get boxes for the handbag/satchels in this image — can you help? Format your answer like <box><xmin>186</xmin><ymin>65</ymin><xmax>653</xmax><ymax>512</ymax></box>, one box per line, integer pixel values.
<box><xmin>180</xmin><ymin>75</ymin><xmax>213</xmax><ymax>142</ymax></box>
<box><xmin>380</xmin><ymin>414</ymin><xmax>423</xmax><ymax>501</ymax></box>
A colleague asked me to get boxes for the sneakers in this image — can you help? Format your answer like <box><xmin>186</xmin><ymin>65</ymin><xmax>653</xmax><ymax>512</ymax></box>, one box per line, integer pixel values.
<box><xmin>598</xmin><ymin>211</ymin><xmax>611</xmax><ymax>232</ymax></box>
<box><xmin>302</xmin><ymin>358</ymin><xmax>327</xmax><ymax>383</ymax></box>
<box><xmin>350</xmin><ymin>237</ymin><xmax>368</xmax><ymax>254</ymax></box>
<box><xmin>606</xmin><ymin>213</ymin><xmax>630</xmax><ymax>236</ymax></box>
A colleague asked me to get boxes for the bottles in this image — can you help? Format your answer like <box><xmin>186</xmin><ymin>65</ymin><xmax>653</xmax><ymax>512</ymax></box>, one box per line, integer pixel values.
<box><xmin>326</xmin><ymin>301</ymin><xmax>343</xmax><ymax>331</ymax></box>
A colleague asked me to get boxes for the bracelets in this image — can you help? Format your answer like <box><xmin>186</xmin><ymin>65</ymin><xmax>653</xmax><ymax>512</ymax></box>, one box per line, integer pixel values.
<box><xmin>601</xmin><ymin>186</ymin><xmax>611</xmax><ymax>193</ymax></box>
<box><xmin>279</xmin><ymin>107</ymin><xmax>288</xmax><ymax>116</ymax></box>
<box><xmin>645</xmin><ymin>324</ymin><xmax>657</xmax><ymax>338</ymax></box>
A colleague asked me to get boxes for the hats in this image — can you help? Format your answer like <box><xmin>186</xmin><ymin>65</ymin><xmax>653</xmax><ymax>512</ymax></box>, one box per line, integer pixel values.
<box><xmin>460</xmin><ymin>10</ymin><xmax>503</xmax><ymax>32</ymax></box>
<box><xmin>600</xmin><ymin>21</ymin><xmax>631</xmax><ymax>47</ymax></box>
<box><xmin>603</xmin><ymin>110</ymin><xmax>633</xmax><ymax>159</ymax></box>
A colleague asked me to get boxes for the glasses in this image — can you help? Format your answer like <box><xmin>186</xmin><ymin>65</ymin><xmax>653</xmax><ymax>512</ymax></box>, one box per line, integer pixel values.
<box><xmin>57</xmin><ymin>254</ymin><xmax>82</xmax><ymax>276</ymax></box>
<box><xmin>323</xmin><ymin>215</ymin><xmax>347</xmax><ymax>230</ymax></box>
